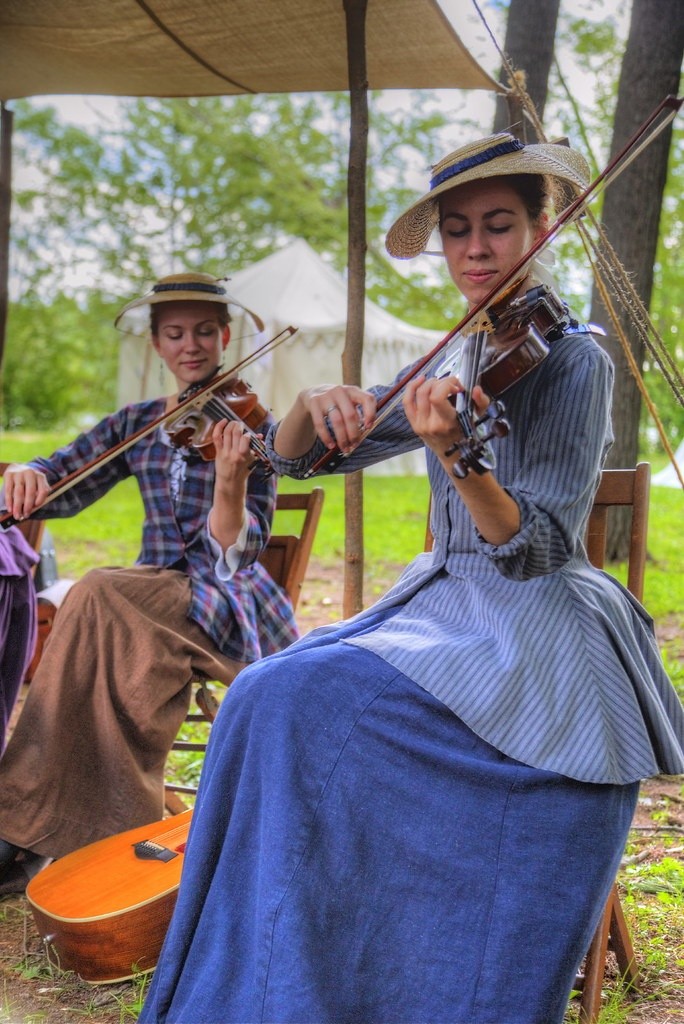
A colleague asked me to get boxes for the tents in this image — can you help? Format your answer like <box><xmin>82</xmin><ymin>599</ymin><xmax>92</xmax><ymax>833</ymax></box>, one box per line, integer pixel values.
<box><xmin>119</xmin><ymin>240</ymin><xmax>445</xmax><ymax>476</ymax></box>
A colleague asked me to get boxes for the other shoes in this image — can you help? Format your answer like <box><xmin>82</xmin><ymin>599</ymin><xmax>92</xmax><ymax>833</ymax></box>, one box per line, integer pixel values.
<box><xmin>0</xmin><ymin>838</ymin><xmax>40</xmax><ymax>896</ymax></box>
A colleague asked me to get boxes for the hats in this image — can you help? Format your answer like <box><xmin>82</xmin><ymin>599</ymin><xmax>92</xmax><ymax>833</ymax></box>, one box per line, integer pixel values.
<box><xmin>112</xmin><ymin>273</ymin><xmax>264</xmax><ymax>343</ymax></box>
<box><xmin>385</xmin><ymin>133</ymin><xmax>591</xmax><ymax>261</ymax></box>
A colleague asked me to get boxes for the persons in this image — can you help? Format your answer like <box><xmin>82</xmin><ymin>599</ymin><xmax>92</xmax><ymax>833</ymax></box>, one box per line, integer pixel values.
<box><xmin>140</xmin><ymin>134</ymin><xmax>683</xmax><ymax>1024</ymax></box>
<box><xmin>0</xmin><ymin>488</ymin><xmax>41</xmax><ymax>756</ymax></box>
<box><xmin>0</xmin><ymin>274</ymin><xmax>298</xmax><ymax>882</ymax></box>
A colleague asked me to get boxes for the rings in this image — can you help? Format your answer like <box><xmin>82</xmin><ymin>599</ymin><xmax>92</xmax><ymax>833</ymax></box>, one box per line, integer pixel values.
<box><xmin>327</xmin><ymin>406</ymin><xmax>336</xmax><ymax>412</ymax></box>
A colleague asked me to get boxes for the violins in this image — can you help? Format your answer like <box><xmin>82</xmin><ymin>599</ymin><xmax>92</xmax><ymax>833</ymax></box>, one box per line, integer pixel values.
<box><xmin>433</xmin><ymin>283</ymin><xmax>570</xmax><ymax>478</ymax></box>
<box><xmin>161</xmin><ymin>374</ymin><xmax>276</xmax><ymax>483</ymax></box>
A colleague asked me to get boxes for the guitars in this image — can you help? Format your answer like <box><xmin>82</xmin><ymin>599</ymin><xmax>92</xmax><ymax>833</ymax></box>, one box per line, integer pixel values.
<box><xmin>24</xmin><ymin>806</ymin><xmax>194</xmax><ymax>985</ymax></box>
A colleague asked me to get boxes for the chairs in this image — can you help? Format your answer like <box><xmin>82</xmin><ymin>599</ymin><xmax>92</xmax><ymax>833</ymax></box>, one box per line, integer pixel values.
<box><xmin>424</xmin><ymin>460</ymin><xmax>651</xmax><ymax>1024</ymax></box>
<box><xmin>164</xmin><ymin>488</ymin><xmax>325</xmax><ymax>814</ymax></box>
